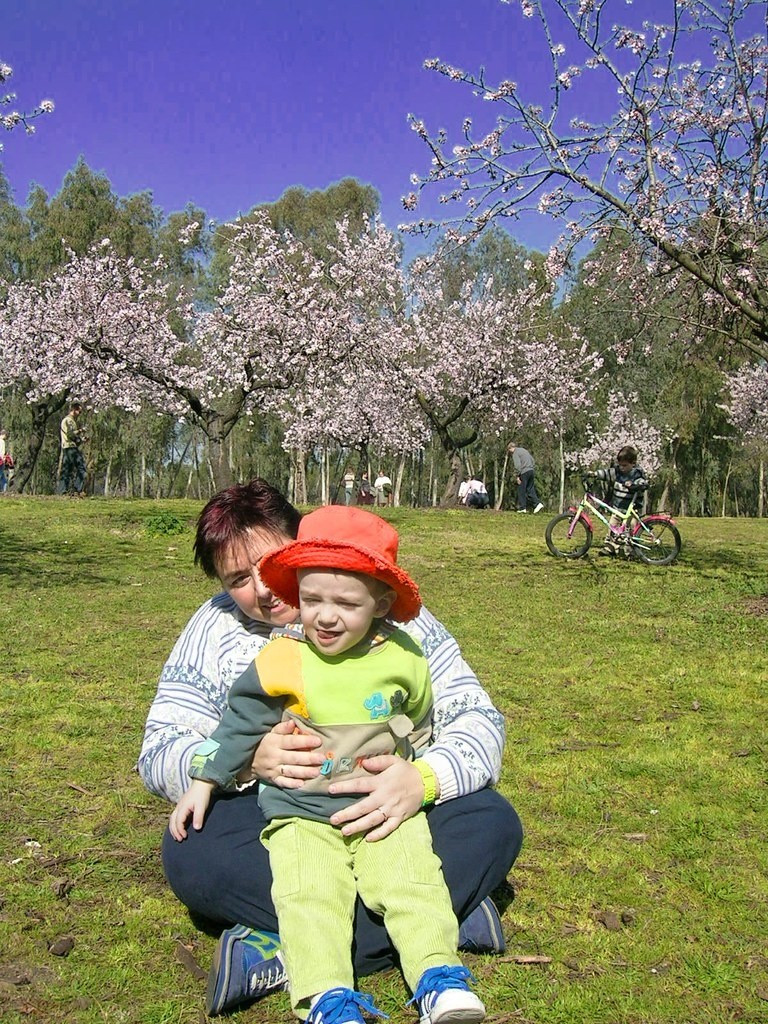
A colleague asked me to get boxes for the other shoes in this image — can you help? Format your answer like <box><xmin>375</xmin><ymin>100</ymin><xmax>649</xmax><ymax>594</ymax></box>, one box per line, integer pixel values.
<box><xmin>625</xmin><ymin>550</ymin><xmax>640</xmax><ymax>562</ymax></box>
<box><xmin>533</xmin><ymin>502</ymin><xmax>544</xmax><ymax>513</ymax></box>
<box><xmin>517</xmin><ymin>508</ymin><xmax>526</xmax><ymax>514</ymax></box>
<box><xmin>599</xmin><ymin>549</ymin><xmax>611</xmax><ymax>555</ymax></box>
<box><xmin>74</xmin><ymin>491</ymin><xmax>85</xmax><ymax>497</ymax></box>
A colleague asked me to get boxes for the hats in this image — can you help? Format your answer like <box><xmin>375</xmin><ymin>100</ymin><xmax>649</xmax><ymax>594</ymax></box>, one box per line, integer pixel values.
<box><xmin>257</xmin><ymin>505</ymin><xmax>422</xmax><ymax>625</ymax></box>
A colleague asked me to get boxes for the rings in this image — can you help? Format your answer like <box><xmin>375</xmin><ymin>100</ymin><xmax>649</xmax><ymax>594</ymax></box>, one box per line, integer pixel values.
<box><xmin>378</xmin><ymin>808</ymin><xmax>390</xmax><ymax>823</ymax></box>
<box><xmin>280</xmin><ymin>765</ymin><xmax>284</xmax><ymax>776</ymax></box>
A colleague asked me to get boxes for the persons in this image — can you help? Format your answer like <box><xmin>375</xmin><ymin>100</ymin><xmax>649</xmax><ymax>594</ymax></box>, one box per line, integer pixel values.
<box><xmin>0</xmin><ymin>429</ymin><xmax>8</xmax><ymax>493</ymax></box>
<box><xmin>507</xmin><ymin>442</ymin><xmax>544</xmax><ymax>514</ymax></box>
<box><xmin>587</xmin><ymin>446</ymin><xmax>649</xmax><ymax>558</ymax></box>
<box><xmin>137</xmin><ymin>466</ymin><xmax>524</xmax><ymax>1024</ymax></box>
<box><xmin>59</xmin><ymin>403</ymin><xmax>90</xmax><ymax>498</ymax></box>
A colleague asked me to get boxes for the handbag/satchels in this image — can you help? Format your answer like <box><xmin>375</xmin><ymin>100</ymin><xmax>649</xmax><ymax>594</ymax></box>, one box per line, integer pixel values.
<box><xmin>468</xmin><ymin>485</ymin><xmax>481</xmax><ymax>504</ymax></box>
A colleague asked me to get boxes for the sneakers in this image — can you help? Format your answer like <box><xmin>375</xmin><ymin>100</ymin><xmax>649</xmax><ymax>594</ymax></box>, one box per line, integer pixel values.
<box><xmin>205</xmin><ymin>924</ymin><xmax>290</xmax><ymax>1017</ymax></box>
<box><xmin>458</xmin><ymin>895</ymin><xmax>505</xmax><ymax>954</ymax></box>
<box><xmin>405</xmin><ymin>966</ymin><xmax>488</xmax><ymax>1024</ymax></box>
<box><xmin>305</xmin><ymin>987</ymin><xmax>389</xmax><ymax>1024</ymax></box>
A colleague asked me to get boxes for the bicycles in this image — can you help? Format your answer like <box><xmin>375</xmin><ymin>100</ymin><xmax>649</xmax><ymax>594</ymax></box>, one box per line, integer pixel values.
<box><xmin>545</xmin><ymin>474</ymin><xmax>682</xmax><ymax>565</ymax></box>
